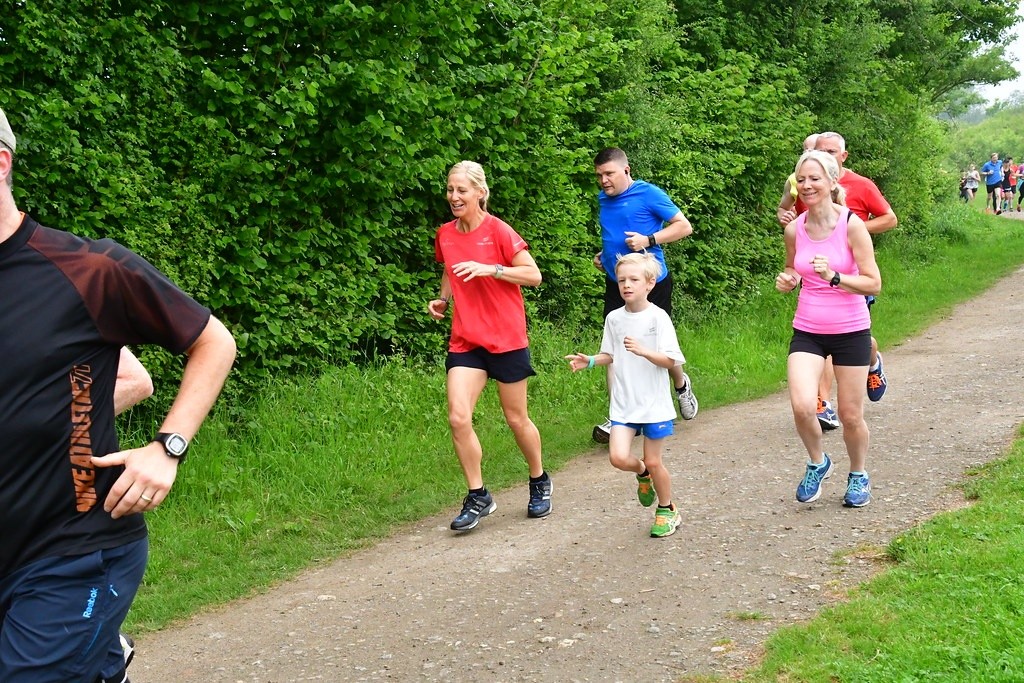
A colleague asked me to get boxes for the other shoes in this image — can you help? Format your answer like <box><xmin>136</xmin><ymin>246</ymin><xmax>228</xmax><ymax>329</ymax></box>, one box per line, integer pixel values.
<box><xmin>1017</xmin><ymin>203</ymin><xmax>1021</xmax><ymax>212</ymax></box>
<box><xmin>986</xmin><ymin>208</ymin><xmax>990</xmax><ymax>214</ymax></box>
<box><xmin>996</xmin><ymin>210</ymin><xmax>1000</xmax><ymax>215</ymax></box>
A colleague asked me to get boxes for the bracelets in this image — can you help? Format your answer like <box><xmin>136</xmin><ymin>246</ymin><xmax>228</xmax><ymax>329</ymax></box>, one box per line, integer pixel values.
<box><xmin>587</xmin><ymin>356</ymin><xmax>594</xmax><ymax>368</ymax></box>
<box><xmin>440</xmin><ymin>298</ymin><xmax>449</xmax><ymax>312</ymax></box>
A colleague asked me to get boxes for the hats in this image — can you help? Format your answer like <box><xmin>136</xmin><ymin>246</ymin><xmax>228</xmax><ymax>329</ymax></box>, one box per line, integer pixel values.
<box><xmin>0</xmin><ymin>109</ymin><xmax>16</xmax><ymax>153</ymax></box>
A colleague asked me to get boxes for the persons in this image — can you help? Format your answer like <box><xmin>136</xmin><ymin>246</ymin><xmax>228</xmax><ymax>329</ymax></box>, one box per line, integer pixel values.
<box><xmin>113</xmin><ymin>345</ymin><xmax>153</xmax><ymax>417</ymax></box>
<box><xmin>794</xmin><ymin>132</ymin><xmax>888</xmax><ymax>430</ymax></box>
<box><xmin>991</xmin><ymin>156</ymin><xmax>1024</xmax><ymax>214</ymax></box>
<box><xmin>777</xmin><ymin>134</ymin><xmax>821</xmax><ymax>219</ymax></box>
<box><xmin>981</xmin><ymin>153</ymin><xmax>1006</xmax><ymax>215</ymax></box>
<box><xmin>564</xmin><ymin>244</ymin><xmax>687</xmax><ymax>538</ymax></box>
<box><xmin>590</xmin><ymin>147</ymin><xmax>699</xmax><ymax>445</ymax></box>
<box><xmin>775</xmin><ymin>152</ymin><xmax>881</xmax><ymax>507</ymax></box>
<box><xmin>958</xmin><ymin>163</ymin><xmax>980</xmax><ymax>204</ymax></box>
<box><xmin>0</xmin><ymin>102</ymin><xmax>238</xmax><ymax>682</ymax></box>
<box><xmin>427</xmin><ymin>158</ymin><xmax>554</xmax><ymax>530</ymax></box>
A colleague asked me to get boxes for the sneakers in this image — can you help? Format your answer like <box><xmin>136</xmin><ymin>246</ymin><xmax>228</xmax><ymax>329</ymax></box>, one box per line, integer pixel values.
<box><xmin>650</xmin><ymin>503</ymin><xmax>681</xmax><ymax>537</ymax></box>
<box><xmin>528</xmin><ymin>471</ymin><xmax>553</xmax><ymax>517</ymax></box>
<box><xmin>636</xmin><ymin>474</ymin><xmax>657</xmax><ymax>507</ymax></box>
<box><xmin>593</xmin><ymin>416</ymin><xmax>641</xmax><ymax>443</ymax></box>
<box><xmin>796</xmin><ymin>452</ymin><xmax>835</xmax><ymax>503</ymax></box>
<box><xmin>451</xmin><ymin>489</ymin><xmax>497</xmax><ymax>530</ymax></box>
<box><xmin>816</xmin><ymin>396</ymin><xmax>839</xmax><ymax>430</ymax></box>
<box><xmin>867</xmin><ymin>351</ymin><xmax>887</xmax><ymax>402</ymax></box>
<box><xmin>842</xmin><ymin>470</ymin><xmax>874</xmax><ymax>507</ymax></box>
<box><xmin>676</xmin><ymin>372</ymin><xmax>698</xmax><ymax>420</ymax></box>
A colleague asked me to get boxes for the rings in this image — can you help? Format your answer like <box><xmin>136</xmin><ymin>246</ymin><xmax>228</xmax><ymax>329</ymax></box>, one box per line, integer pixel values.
<box><xmin>141</xmin><ymin>495</ymin><xmax>152</xmax><ymax>503</ymax></box>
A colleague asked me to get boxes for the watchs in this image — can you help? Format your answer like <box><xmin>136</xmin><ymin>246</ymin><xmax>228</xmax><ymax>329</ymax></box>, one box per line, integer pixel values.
<box><xmin>152</xmin><ymin>432</ymin><xmax>189</xmax><ymax>464</ymax></box>
<box><xmin>830</xmin><ymin>271</ymin><xmax>840</xmax><ymax>287</ymax></box>
<box><xmin>647</xmin><ymin>234</ymin><xmax>656</xmax><ymax>247</ymax></box>
<box><xmin>494</xmin><ymin>264</ymin><xmax>503</xmax><ymax>279</ymax></box>
